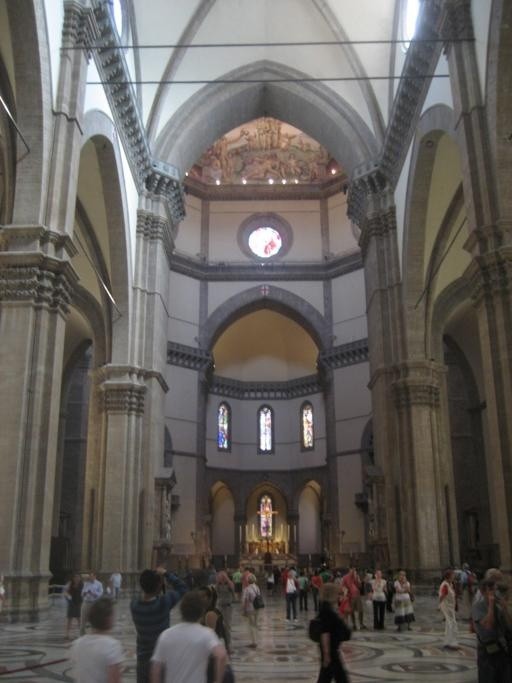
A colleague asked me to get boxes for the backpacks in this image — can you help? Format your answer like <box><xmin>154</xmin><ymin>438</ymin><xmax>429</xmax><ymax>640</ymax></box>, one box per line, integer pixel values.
<box><xmin>251</xmin><ymin>586</ymin><xmax>265</xmax><ymax>609</ymax></box>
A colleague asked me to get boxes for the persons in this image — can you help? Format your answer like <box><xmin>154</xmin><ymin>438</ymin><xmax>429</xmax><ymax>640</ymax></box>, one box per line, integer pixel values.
<box><xmin>472</xmin><ymin>567</ymin><xmax>511</xmax><ymax>640</ymax></box>
<box><xmin>64</xmin><ymin>573</ymin><xmax>87</xmax><ymax>638</ymax></box>
<box><xmin>317</xmin><ymin>582</ymin><xmax>352</xmax><ymax>682</ymax></box>
<box><xmin>471</xmin><ymin>578</ymin><xmax>511</xmax><ymax>683</ymax></box>
<box><xmin>198</xmin><ymin>587</ymin><xmax>229</xmax><ymax>681</ymax></box>
<box><xmin>149</xmin><ymin>589</ymin><xmax>230</xmax><ymax>682</ymax></box>
<box><xmin>70</xmin><ymin>597</ymin><xmax>124</xmax><ymax>683</ymax></box>
<box><xmin>129</xmin><ymin>567</ymin><xmax>185</xmax><ymax>682</ymax></box>
<box><xmin>206</xmin><ymin>557</ymin><xmax>479</xmax><ymax>651</ymax></box>
<box><xmin>110</xmin><ymin>568</ymin><xmax>123</xmax><ymax>602</ymax></box>
<box><xmin>79</xmin><ymin>573</ymin><xmax>104</xmax><ymax>637</ymax></box>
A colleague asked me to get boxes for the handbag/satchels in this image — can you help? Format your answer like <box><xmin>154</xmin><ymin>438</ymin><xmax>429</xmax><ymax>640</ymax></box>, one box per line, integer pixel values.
<box><xmin>309</xmin><ymin>618</ymin><xmax>352</xmax><ymax>643</ymax></box>
<box><xmin>483</xmin><ymin>639</ymin><xmax>507</xmax><ymax>667</ymax></box>
